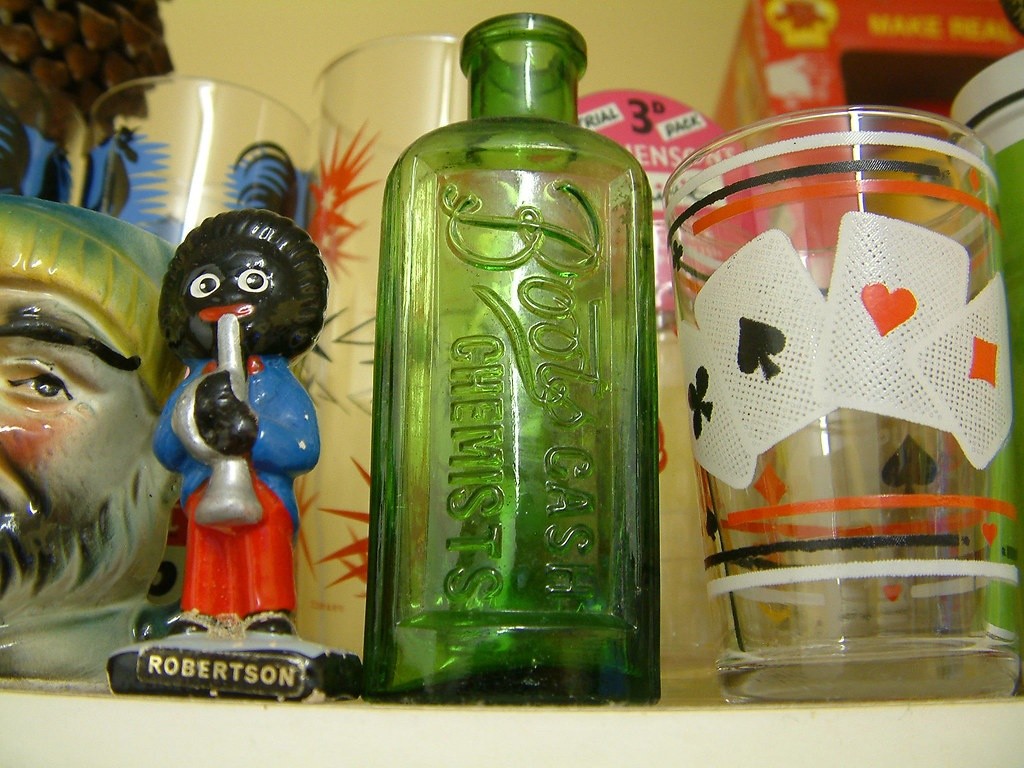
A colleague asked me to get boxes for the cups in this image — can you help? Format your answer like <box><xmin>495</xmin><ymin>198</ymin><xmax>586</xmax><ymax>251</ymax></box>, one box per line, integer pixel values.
<box><xmin>950</xmin><ymin>49</ymin><xmax>1024</xmax><ymax>642</ymax></box>
<box><xmin>0</xmin><ymin>194</ymin><xmax>232</xmax><ymax>676</ymax></box>
<box><xmin>87</xmin><ymin>77</ymin><xmax>311</xmax><ymax>244</ymax></box>
<box><xmin>0</xmin><ymin>67</ymin><xmax>85</xmax><ymax>211</ymax></box>
<box><xmin>663</xmin><ymin>106</ymin><xmax>1018</xmax><ymax>699</ymax></box>
<box><xmin>295</xmin><ymin>33</ymin><xmax>466</xmax><ymax>658</ymax></box>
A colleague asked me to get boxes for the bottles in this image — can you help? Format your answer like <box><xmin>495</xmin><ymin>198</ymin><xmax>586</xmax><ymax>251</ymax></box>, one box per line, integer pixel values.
<box><xmin>360</xmin><ymin>12</ymin><xmax>661</xmax><ymax>704</ymax></box>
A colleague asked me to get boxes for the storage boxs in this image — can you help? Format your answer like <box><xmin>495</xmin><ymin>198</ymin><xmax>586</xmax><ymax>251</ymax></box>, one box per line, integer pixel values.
<box><xmin>709</xmin><ymin>0</ymin><xmax>1024</xmax><ymax>251</ymax></box>
